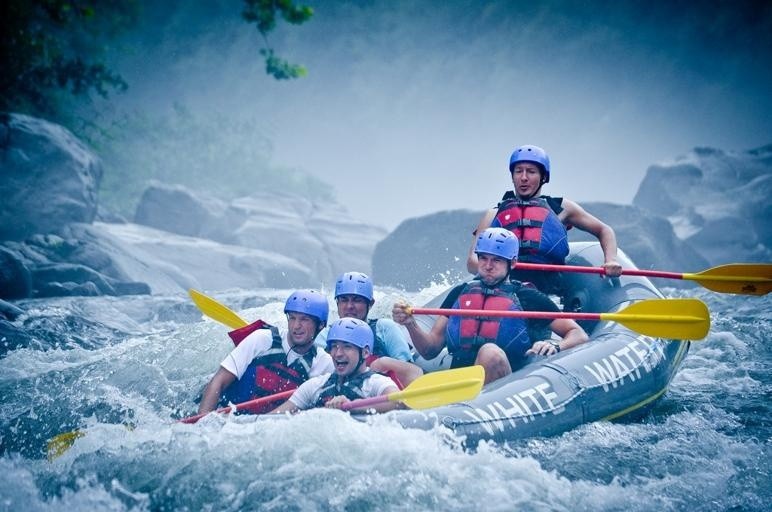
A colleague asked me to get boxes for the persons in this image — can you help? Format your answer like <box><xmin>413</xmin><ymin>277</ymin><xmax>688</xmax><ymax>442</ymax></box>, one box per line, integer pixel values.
<box><xmin>313</xmin><ymin>272</ymin><xmax>424</xmax><ymax>386</ymax></box>
<box><xmin>394</xmin><ymin>227</ymin><xmax>589</xmax><ymax>386</ymax></box>
<box><xmin>196</xmin><ymin>288</ymin><xmax>334</xmax><ymax>419</ymax></box>
<box><xmin>467</xmin><ymin>143</ymin><xmax>623</xmax><ymax>310</ymax></box>
<box><xmin>267</xmin><ymin>317</ymin><xmax>403</xmax><ymax>417</ymax></box>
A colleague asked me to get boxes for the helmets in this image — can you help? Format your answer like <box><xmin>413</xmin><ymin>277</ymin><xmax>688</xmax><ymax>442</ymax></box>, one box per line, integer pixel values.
<box><xmin>475</xmin><ymin>227</ymin><xmax>520</xmax><ymax>260</ymax></box>
<box><xmin>326</xmin><ymin>317</ymin><xmax>374</xmax><ymax>355</ymax></box>
<box><xmin>334</xmin><ymin>271</ymin><xmax>372</xmax><ymax>301</ymax></box>
<box><xmin>509</xmin><ymin>145</ymin><xmax>550</xmax><ymax>184</ymax></box>
<box><xmin>284</xmin><ymin>289</ymin><xmax>329</xmax><ymax>327</ymax></box>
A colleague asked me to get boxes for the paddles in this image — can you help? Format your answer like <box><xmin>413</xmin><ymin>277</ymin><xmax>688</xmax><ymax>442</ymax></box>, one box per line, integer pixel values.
<box><xmin>399</xmin><ymin>297</ymin><xmax>710</xmax><ymax>340</ymax></box>
<box><xmin>49</xmin><ymin>389</ymin><xmax>295</xmax><ymax>465</ymax></box>
<box><xmin>189</xmin><ymin>288</ymin><xmax>249</xmax><ymax>329</ymax></box>
<box><xmin>515</xmin><ymin>262</ymin><xmax>772</xmax><ymax>295</ymax></box>
<box><xmin>340</xmin><ymin>365</ymin><xmax>486</xmax><ymax>411</ymax></box>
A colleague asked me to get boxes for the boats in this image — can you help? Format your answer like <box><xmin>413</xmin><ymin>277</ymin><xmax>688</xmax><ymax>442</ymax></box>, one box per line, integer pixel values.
<box><xmin>208</xmin><ymin>240</ymin><xmax>692</xmax><ymax>459</ymax></box>
